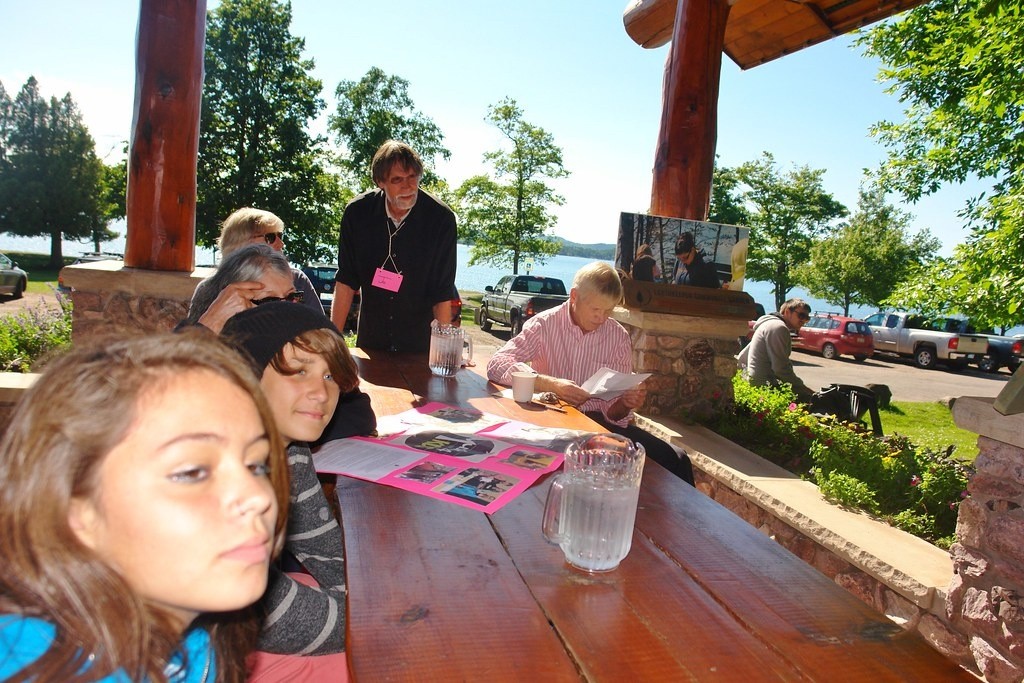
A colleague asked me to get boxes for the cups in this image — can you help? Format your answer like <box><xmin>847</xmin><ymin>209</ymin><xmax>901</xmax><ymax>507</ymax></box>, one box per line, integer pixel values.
<box><xmin>511</xmin><ymin>371</ymin><xmax>537</xmax><ymax>403</ymax></box>
<box><xmin>429</xmin><ymin>323</ymin><xmax>473</xmax><ymax>377</ymax></box>
<box><xmin>541</xmin><ymin>432</ymin><xmax>645</xmax><ymax>572</ymax></box>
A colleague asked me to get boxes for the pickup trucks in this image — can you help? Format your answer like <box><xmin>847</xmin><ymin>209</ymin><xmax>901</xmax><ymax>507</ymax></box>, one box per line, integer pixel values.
<box><xmin>479</xmin><ymin>274</ymin><xmax>571</xmax><ymax>339</ymax></box>
<box><xmin>930</xmin><ymin>316</ymin><xmax>1024</xmax><ymax>373</ymax></box>
<box><xmin>858</xmin><ymin>312</ymin><xmax>988</xmax><ymax>370</ymax></box>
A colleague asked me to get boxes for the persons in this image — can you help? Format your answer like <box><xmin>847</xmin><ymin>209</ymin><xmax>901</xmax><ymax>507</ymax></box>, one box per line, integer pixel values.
<box><xmin>0</xmin><ymin>331</ymin><xmax>288</xmax><ymax>683</ymax></box>
<box><xmin>631</xmin><ymin>232</ymin><xmax>748</xmax><ymax>290</ymax></box>
<box><xmin>186</xmin><ymin>208</ymin><xmax>325</xmax><ymax>317</ymax></box>
<box><xmin>221</xmin><ymin>301</ymin><xmax>359</xmax><ymax>683</ymax></box>
<box><xmin>738</xmin><ymin>299</ymin><xmax>814</xmax><ymax>397</ymax></box>
<box><xmin>331</xmin><ymin>141</ymin><xmax>457</xmax><ymax>350</ymax></box>
<box><xmin>487</xmin><ymin>262</ymin><xmax>695</xmax><ymax>488</ymax></box>
<box><xmin>198</xmin><ymin>244</ymin><xmax>378</xmax><ymax>447</ymax></box>
<box><xmin>402</xmin><ymin>409</ymin><xmax>572</xmax><ymax>506</ymax></box>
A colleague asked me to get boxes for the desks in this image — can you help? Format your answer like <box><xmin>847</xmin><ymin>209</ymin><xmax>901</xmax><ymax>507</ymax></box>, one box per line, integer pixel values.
<box><xmin>336</xmin><ymin>345</ymin><xmax>983</xmax><ymax>683</ymax></box>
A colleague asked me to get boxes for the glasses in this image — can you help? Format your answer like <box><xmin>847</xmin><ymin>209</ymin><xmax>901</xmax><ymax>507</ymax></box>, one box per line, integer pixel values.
<box><xmin>252</xmin><ymin>232</ymin><xmax>284</xmax><ymax>244</ymax></box>
<box><xmin>384</xmin><ymin>173</ymin><xmax>422</xmax><ymax>186</ymax></box>
<box><xmin>675</xmin><ymin>247</ymin><xmax>694</xmax><ymax>263</ymax></box>
<box><xmin>792</xmin><ymin>308</ymin><xmax>809</xmax><ymax>323</ymax></box>
<box><xmin>242</xmin><ymin>290</ymin><xmax>305</xmax><ymax>306</ymax></box>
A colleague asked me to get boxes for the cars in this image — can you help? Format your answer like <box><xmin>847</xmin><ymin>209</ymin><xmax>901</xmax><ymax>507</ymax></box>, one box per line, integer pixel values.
<box><xmin>0</xmin><ymin>252</ymin><xmax>28</xmax><ymax>299</ymax></box>
<box><xmin>299</xmin><ymin>260</ymin><xmax>361</xmax><ymax>331</ymax></box>
<box><xmin>57</xmin><ymin>251</ymin><xmax>124</xmax><ymax>292</ymax></box>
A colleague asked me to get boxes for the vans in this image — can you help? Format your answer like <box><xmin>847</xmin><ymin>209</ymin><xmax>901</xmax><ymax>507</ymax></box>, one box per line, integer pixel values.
<box><xmin>790</xmin><ymin>311</ymin><xmax>874</xmax><ymax>362</ymax></box>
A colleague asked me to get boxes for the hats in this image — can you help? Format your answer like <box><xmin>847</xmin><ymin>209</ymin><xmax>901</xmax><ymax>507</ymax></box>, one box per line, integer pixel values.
<box><xmin>218</xmin><ymin>300</ymin><xmax>347</xmax><ymax>380</ymax></box>
